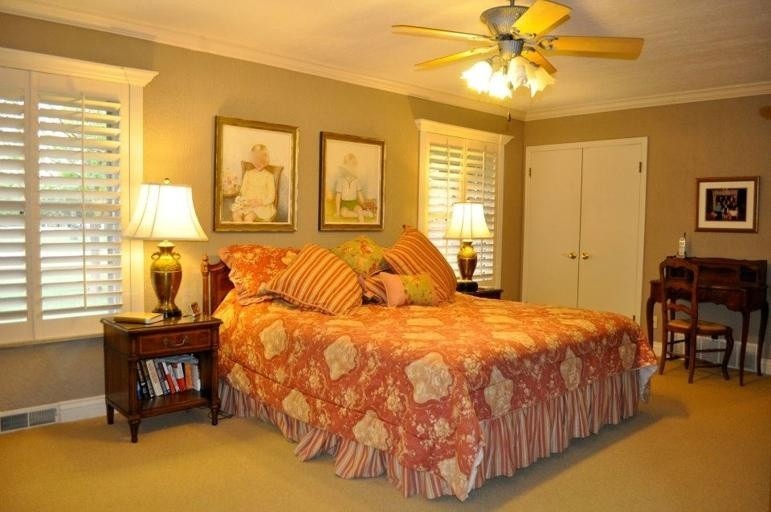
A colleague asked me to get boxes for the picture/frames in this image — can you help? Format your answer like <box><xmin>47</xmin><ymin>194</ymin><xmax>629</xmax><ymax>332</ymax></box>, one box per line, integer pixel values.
<box><xmin>696</xmin><ymin>174</ymin><xmax>759</xmax><ymax>232</ymax></box>
<box><xmin>318</xmin><ymin>131</ymin><xmax>386</xmax><ymax>229</ymax></box>
<box><xmin>216</xmin><ymin>116</ymin><xmax>299</xmax><ymax>231</ymax></box>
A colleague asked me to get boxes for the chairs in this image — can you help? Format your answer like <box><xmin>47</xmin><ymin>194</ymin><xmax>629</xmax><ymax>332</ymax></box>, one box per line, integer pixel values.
<box><xmin>658</xmin><ymin>258</ymin><xmax>734</xmax><ymax>382</ymax></box>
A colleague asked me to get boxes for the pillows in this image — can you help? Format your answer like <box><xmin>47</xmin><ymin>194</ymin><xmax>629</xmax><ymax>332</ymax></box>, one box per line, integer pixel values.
<box><xmin>269</xmin><ymin>244</ymin><xmax>363</xmax><ymax>315</ymax></box>
<box><xmin>379</xmin><ymin>272</ymin><xmax>441</xmax><ymax>309</ymax></box>
<box><xmin>220</xmin><ymin>245</ymin><xmax>319</xmax><ymax>306</ymax></box>
<box><xmin>335</xmin><ymin>234</ymin><xmax>393</xmax><ymax>278</ymax></box>
<box><xmin>362</xmin><ymin>271</ymin><xmax>387</xmax><ymax>303</ymax></box>
<box><xmin>383</xmin><ymin>224</ymin><xmax>460</xmax><ymax>305</ymax></box>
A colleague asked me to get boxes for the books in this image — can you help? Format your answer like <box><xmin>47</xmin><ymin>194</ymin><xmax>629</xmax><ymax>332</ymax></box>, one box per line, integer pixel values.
<box><xmin>113</xmin><ymin>310</ymin><xmax>165</xmax><ymax>324</ymax></box>
<box><xmin>134</xmin><ymin>354</ymin><xmax>202</xmax><ymax>397</ymax></box>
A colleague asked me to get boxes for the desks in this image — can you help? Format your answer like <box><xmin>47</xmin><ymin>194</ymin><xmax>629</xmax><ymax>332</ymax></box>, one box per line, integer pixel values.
<box><xmin>642</xmin><ymin>258</ymin><xmax>770</xmax><ymax>385</ymax></box>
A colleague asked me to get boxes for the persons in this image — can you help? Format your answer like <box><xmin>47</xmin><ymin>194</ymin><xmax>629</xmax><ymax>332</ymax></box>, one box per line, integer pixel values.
<box><xmin>715</xmin><ymin>196</ymin><xmax>740</xmax><ymax>221</ymax></box>
<box><xmin>232</xmin><ymin>141</ymin><xmax>278</xmax><ymax>224</ymax></box>
<box><xmin>331</xmin><ymin>152</ymin><xmax>378</xmax><ymax>223</ymax></box>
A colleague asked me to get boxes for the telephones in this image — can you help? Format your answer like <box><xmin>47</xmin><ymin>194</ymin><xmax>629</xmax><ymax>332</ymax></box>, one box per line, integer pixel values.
<box><xmin>678</xmin><ymin>233</ymin><xmax>686</xmax><ymax>259</ymax></box>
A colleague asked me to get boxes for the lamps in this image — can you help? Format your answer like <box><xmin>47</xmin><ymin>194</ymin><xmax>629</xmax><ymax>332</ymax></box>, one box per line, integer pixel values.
<box><xmin>460</xmin><ymin>1</ymin><xmax>558</xmax><ymax>101</ymax></box>
<box><xmin>441</xmin><ymin>200</ymin><xmax>492</xmax><ymax>292</ymax></box>
<box><xmin>122</xmin><ymin>182</ymin><xmax>209</xmax><ymax>314</ymax></box>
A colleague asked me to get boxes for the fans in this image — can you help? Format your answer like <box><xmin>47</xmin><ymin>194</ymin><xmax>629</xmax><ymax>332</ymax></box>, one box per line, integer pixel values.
<box><xmin>389</xmin><ymin>1</ymin><xmax>646</xmax><ymax>77</ymax></box>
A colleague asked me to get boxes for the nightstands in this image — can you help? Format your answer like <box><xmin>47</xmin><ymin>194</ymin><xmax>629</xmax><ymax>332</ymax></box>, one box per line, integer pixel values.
<box><xmin>461</xmin><ymin>283</ymin><xmax>505</xmax><ymax>301</ymax></box>
<box><xmin>103</xmin><ymin>316</ymin><xmax>227</xmax><ymax>441</ymax></box>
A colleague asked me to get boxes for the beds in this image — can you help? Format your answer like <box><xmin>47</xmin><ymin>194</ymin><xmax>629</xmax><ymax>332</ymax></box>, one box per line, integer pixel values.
<box><xmin>199</xmin><ymin>246</ymin><xmax>658</xmax><ymax>502</ymax></box>
